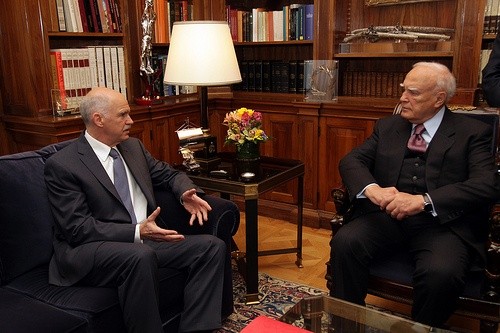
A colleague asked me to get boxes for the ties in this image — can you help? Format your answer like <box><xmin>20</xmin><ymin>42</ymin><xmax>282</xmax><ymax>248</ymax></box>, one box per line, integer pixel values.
<box><xmin>407</xmin><ymin>124</ymin><xmax>426</xmax><ymax>152</ymax></box>
<box><xmin>109</xmin><ymin>148</ymin><xmax>137</xmax><ymax>224</ymax></box>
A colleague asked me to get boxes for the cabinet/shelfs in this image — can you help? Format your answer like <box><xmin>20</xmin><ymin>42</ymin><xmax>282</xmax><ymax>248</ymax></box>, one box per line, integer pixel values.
<box><xmin>0</xmin><ymin>0</ymin><xmax>488</xmax><ymax>230</ymax></box>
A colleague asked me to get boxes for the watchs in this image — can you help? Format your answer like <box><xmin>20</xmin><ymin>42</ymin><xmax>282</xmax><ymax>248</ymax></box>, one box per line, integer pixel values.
<box><xmin>423</xmin><ymin>193</ymin><xmax>432</xmax><ymax>212</ymax></box>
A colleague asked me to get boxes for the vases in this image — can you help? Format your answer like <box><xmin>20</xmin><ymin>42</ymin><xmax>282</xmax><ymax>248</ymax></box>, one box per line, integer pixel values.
<box><xmin>238</xmin><ymin>142</ymin><xmax>260</xmax><ymax>161</ymax></box>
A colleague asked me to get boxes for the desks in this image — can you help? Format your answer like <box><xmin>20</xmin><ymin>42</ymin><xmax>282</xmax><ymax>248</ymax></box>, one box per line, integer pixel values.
<box><xmin>174</xmin><ymin>151</ymin><xmax>304</xmax><ymax>305</ymax></box>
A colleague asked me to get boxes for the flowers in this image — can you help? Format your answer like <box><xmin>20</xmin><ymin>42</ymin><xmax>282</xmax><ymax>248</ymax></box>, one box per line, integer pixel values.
<box><xmin>226</xmin><ymin>107</ymin><xmax>265</xmax><ymax>142</ymax></box>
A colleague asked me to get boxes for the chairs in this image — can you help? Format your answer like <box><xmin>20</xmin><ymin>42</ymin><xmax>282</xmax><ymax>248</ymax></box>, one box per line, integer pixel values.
<box><xmin>0</xmin><ymin>138</ymin><xmax>240</xmax><ymax>333</ymax></box>
<box><xmin>325</xmin><ymin>102</ymin><xmax>500</xmax><ymax>333</ymax></box>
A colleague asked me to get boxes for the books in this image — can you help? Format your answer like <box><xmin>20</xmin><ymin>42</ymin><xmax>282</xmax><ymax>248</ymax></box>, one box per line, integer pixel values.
<box><xmin>343</xmin><ymin>69</ymin><xmax>408</xmax><ymax>98</ymax></box>
<box><xmin>50</xmin><ymin>46</ymin><xmax>127</xmax><ymax>113</ymax></box>
<box><xmin>152</xmin><ymin>54</ymin><xmax>197</xmax><ymax>97</ymax></box>
<box><xmin>44</xmin><ymin>0</ymin><xmax>122</xmax><ymax>33</ymax></box>
<box><xmin>478</xmin><ymin>15</ymin><xmax>500</xmax><ymax>84</ymax></box>
<box><xmin>152</xmin><ymin>0</ymin><xmax>314</xmax><ymax>42</ymax></box>
<box><xmin>232</xmin><ymin>59</ymin><xmax>312</xmax><ymax>94</ymax></box>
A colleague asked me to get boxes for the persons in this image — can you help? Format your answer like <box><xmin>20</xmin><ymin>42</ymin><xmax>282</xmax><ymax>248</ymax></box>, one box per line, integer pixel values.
<box><xmin>328</xmin><ymin>60</ymin><xmax>500</xmax><ymax>333</ymax></box>
<box><xmin>481</xmin><ymin>32</ymin><xmax>500</xmax><ymax>108</ymax></box>
<box><xmin>44</xmin><ymin>87</ymin><xmax>227</xmax><ymax>333</ymax></box>
